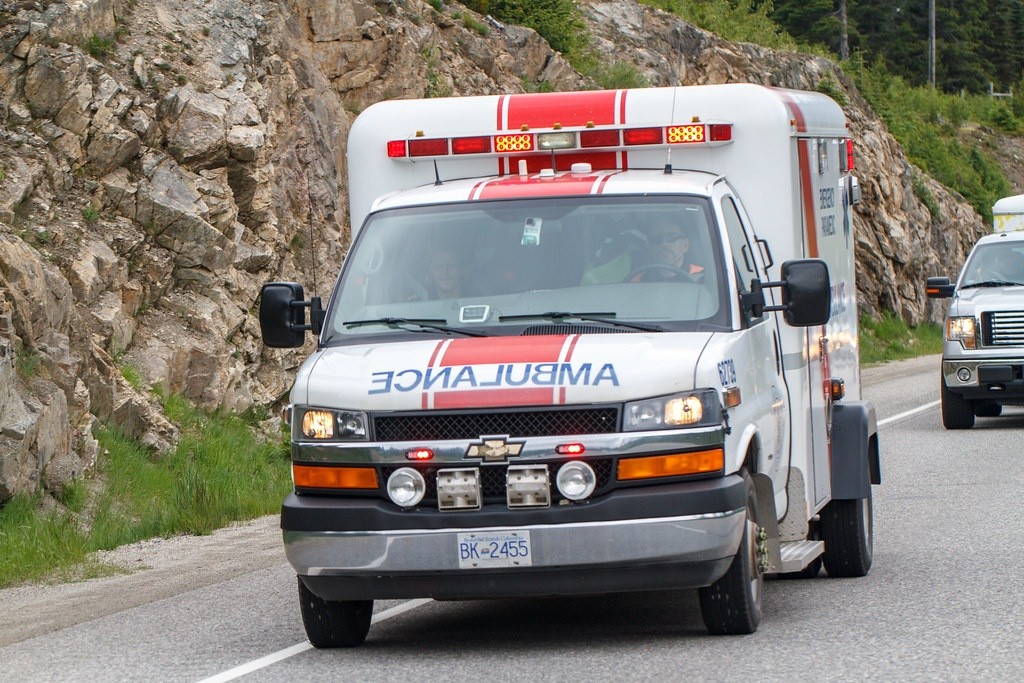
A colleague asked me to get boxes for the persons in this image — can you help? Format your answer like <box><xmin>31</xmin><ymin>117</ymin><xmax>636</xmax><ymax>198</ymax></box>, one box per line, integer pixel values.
<box><xmin>631</xmin><ymin>218</ymin><xmax>704</xmax><ymax>284</ymax></box>
<box><xmin>405</xmin><ymin>247</ymin><xmax>466</xmax><ymax>302</ymax></box>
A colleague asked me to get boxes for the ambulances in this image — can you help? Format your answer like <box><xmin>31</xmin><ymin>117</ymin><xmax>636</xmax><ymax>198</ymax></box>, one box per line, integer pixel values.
<box><xmin>255</xmin><ymin>84</ymin><xmax>882</xmax><ymax>649</ymax></box>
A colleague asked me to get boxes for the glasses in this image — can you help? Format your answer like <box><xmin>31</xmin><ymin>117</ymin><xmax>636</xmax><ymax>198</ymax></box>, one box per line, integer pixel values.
<box><xmin>652</xmin><ymin>233</ymin><xmax>681</xmax><ymax>244</ymax></box>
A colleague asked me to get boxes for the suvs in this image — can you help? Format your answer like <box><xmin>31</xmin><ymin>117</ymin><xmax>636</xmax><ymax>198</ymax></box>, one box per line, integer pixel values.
<box><xmin>925</xmin><ymin>232</ymin><xmax>1024</xmax><ymax>427</ymax></box>
